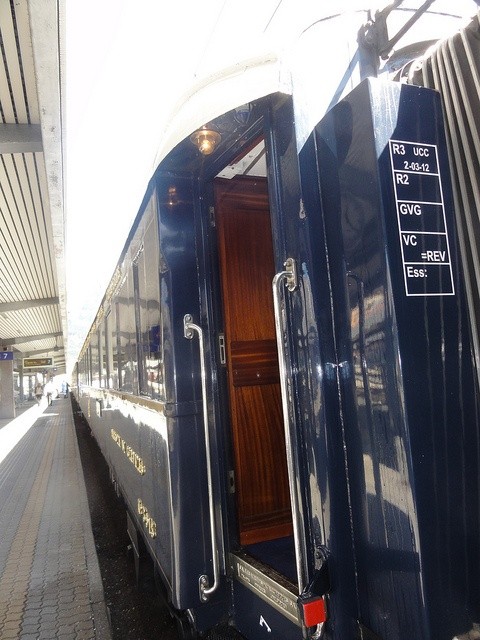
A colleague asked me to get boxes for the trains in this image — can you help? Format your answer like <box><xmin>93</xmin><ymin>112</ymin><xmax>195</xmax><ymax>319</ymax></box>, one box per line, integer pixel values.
<box><xmin>71</xmin><ymin>13</ymin><xmax>479</xmax><ymax>640</ymax></box>
